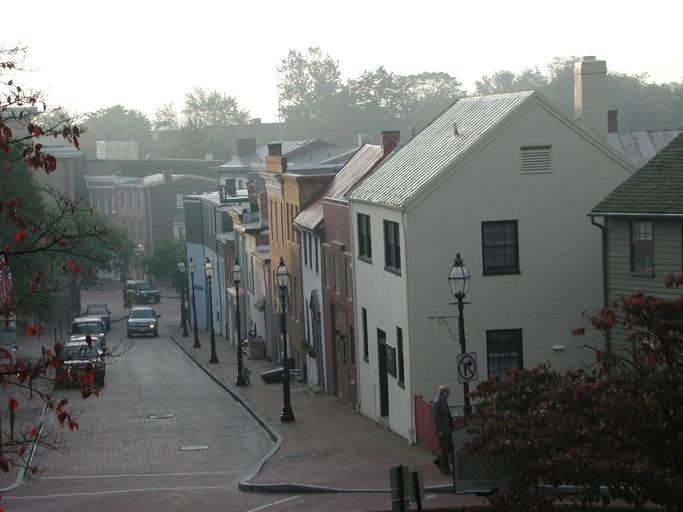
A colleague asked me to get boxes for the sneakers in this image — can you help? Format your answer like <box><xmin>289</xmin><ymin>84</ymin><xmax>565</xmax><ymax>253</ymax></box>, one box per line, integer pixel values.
<box><xmin>433</xmin><ymin>458</ymin><xmax>440</xmax><ymax>470</ymax></box>
<box><xmin>440</xmin><ymin>464</ymin><xmax>453</xmax><ymax>473</ymax></box>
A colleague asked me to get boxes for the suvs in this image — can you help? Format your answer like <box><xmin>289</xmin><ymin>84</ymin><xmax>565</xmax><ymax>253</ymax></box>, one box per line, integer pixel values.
<box><xmin>125</xmin><ymin>279</ymin><xmax>161</xmax><ymax>303</ymax></box>
<box><xmin>126</xmin><ymin>306</ymin><xmax>160</xmax><ymax>338</ymax></box>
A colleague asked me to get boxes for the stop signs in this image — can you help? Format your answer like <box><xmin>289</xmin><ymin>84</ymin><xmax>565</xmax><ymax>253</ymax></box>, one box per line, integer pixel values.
<box><xmin>1</xmin><ymin>346</ymin><xmax>16</xmax><ymax>375</ymax></box>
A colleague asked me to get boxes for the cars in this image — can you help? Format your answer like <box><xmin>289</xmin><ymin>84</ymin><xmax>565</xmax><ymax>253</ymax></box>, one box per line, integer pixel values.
<box><xmin>56</xmin><ymin>303</ymin><xmax>112</xmax><ymax>387</ymax></box>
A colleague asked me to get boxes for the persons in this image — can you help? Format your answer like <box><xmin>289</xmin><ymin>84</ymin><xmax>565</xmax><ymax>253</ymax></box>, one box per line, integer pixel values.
<box><xmin>431</xmin><ymin>384</ymin><xmax>454</xmax><ymax>474</ymax></box>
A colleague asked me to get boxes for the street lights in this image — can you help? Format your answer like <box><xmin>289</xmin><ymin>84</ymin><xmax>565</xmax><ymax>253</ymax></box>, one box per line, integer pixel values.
<box><xmin>230</xmin><ymin>257</ymin><xmax>248</xmax><ymax>386</ymax></box>
<box><xmin>448</xmin><ymin>252</ymin><xmax>474</xmax><ymax>426</ymax></box>
<box><xmin>139</xmin><ymin>240</ymin><xmax>145</xmax><ymax>282</ymax></box>
<box><xmin>203</xmin><ymin>256</ymin><xmax>219</xmax><ymax>363</ymax></box>
<box><xmin>275</xmin><ymin>257</ymin><xmax>295</xmax><ymax>421</ymax></box>
<box><xmin>187</xmin><ymin>256</ymin><xmax>201</xmax><ymax>349</ymax></box>
<box><xmin>177</xmin><ymin>257</ymin><xmax>188</xmax><ymax>337</ymax></box>
